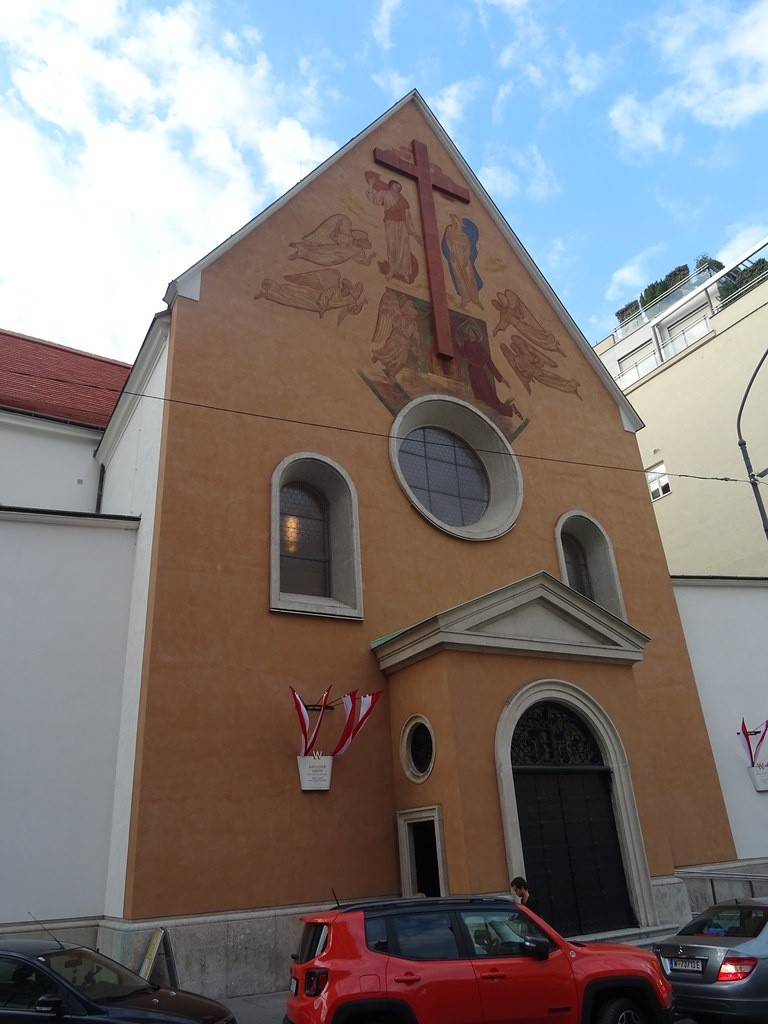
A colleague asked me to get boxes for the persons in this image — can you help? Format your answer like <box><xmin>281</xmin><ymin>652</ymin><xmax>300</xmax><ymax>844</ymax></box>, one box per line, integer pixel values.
<box><xmin>511</xmin><ymin>875</ymin><xmax>541</xmax><ymax>933</ymax></box>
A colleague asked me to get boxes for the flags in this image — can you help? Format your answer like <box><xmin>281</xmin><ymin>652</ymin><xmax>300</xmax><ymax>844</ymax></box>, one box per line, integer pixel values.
<box><xmin>291</xmin><ymin>688</ymin><xmax>384</xmax><ymax>756</ymax></box>
<box><xmin>740</xmin><ymin>718</ymin><xmax>768</xmax><ymax>768</ymax></box>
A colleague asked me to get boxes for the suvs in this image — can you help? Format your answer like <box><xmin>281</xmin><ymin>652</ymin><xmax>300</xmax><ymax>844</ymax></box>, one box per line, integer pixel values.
<box><xmin>283</xmin><ymin>896</ymin><xmax>678</xmax><ymax>1024</ymax></box>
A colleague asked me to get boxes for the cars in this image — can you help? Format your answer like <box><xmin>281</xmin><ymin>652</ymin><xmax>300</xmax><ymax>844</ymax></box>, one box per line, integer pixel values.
<box><xmin>1</xmin><ymin>938</ymin><xmax>241</xmax><ymax>1024</ymax></box>
<box><xmin>652</xmin><ymin>897</ymin><xmax>768</xmax><ymax>1024</ymax></box>
